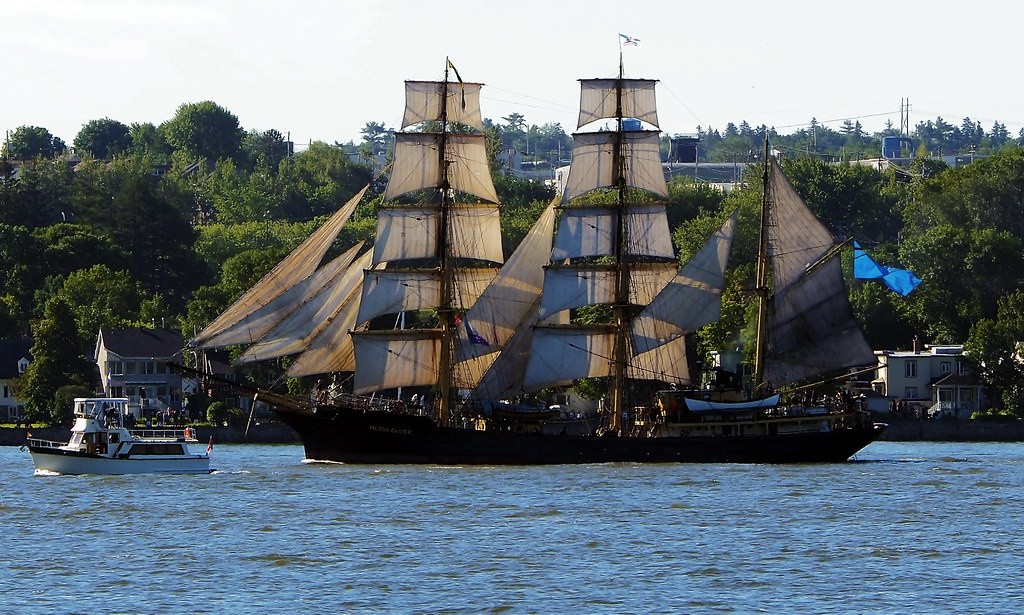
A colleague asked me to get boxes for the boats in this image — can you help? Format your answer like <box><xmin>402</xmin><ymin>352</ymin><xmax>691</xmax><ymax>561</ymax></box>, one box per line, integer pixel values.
<box><xmin>23</xmin><ymin>390</ymin><xmax>214</xmax><ymax>477</ymax></box>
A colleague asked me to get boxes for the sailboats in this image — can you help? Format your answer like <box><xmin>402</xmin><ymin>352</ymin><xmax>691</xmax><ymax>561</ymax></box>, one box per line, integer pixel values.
<box><xmin>164</xmin><ymin>32</ymin><xmax>919</xmax><ymax>466</ymax></box>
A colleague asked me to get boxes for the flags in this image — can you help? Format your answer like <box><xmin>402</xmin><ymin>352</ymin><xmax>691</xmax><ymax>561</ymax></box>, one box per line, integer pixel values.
<box><xmin>852</xmin><ymin>240</ymin><xmax>922</xmax><ymax>297</ymax></box>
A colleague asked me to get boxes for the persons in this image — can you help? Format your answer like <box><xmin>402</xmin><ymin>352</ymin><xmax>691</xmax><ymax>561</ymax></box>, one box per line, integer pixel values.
<box><xmin>397</xmin><ymin>393</ymin><xmax>425</xmax><ymax>415</ymax></box>
<box><xmin>14</xmin><ymin>416</ymin><xmax>31</xmax><ymax>428</ymax></box>
<box><xmin>154</xmin><ymin>406</ymin><xmax>169</xmax><ymax>426</ymax></box>
<box><xmin>813</xmin><ymin>390</ymin><xmax>868</xmax><ymax>431</ymax></box>
<box><xmin>596</xmin><ymin>422</ymin><xmax>642</xmax><ymax>440</ymax></box>
<box><xmin>311</xmin><ymin>380</ymin><xmax>336</xmax><ymax>407</ymax></box>
